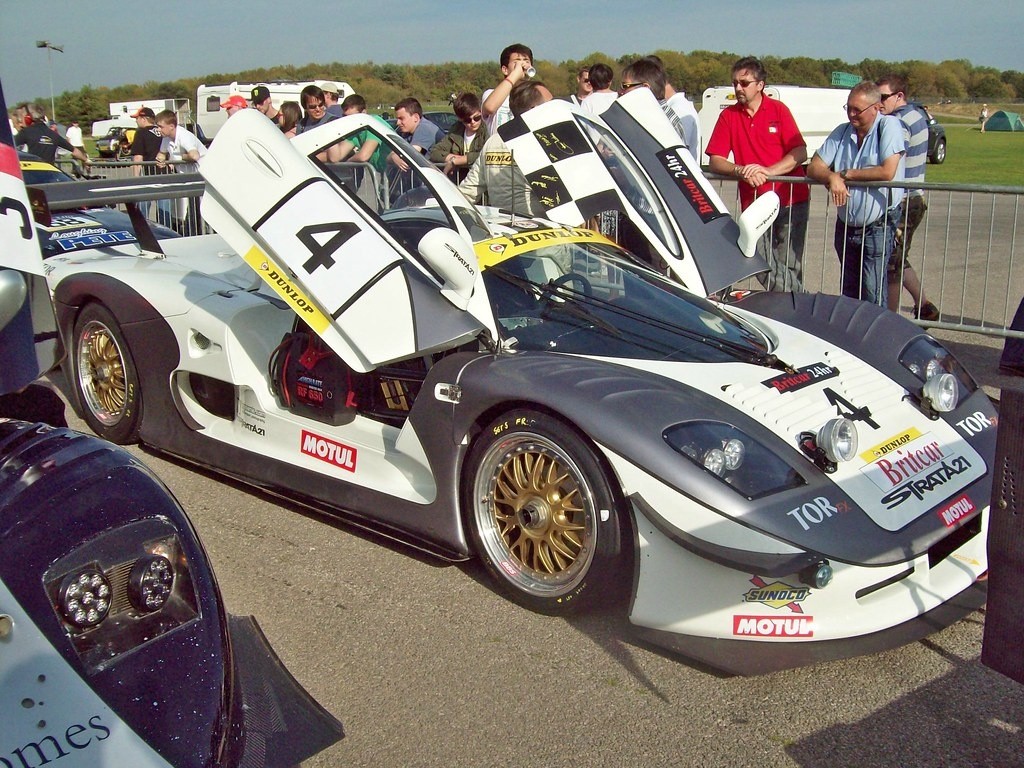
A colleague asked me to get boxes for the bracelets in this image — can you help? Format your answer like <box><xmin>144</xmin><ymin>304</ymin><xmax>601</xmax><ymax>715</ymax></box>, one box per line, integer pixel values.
<box><xmin>504</xmin><ymin>78</ymin><xmax>514</xmax><ymax>87</ymax></box>
<box><xmin>734</xmin><ymin>165</ymin><xmax>744</xmax><ymax>177</ymax></box>
<box><xmin>391</xmin><ymin>153</ymin><xmax>394</xmax><ymax>159</ymax></box>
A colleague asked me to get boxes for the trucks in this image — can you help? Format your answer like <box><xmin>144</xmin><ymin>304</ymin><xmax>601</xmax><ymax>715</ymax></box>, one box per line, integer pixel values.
<box><xmin>196</xmin><ymin>80</ymin><xmax>356</xmax><ymax>142</ymax></box>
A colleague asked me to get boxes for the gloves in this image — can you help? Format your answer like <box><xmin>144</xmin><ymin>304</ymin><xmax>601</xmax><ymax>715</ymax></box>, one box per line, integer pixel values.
<box><xmin>85</xmin><ymin>158</ymin><xmax>93</xmax><ymax>167</ymax></box>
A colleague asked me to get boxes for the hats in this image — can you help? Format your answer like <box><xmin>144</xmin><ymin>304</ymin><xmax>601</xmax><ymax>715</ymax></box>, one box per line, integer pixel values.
<box><xmin>50</xmin><ymin>121</ymin><xmax>57</xmax><ymax>126</ymax></box>
<box><xmin>246</xmin><ymin>86</ymin><xmax>270</xmax><ymax>102</ymax></box>
<box><xmin>130</xmin><ymin>107</ymin><xmax>155</xmax><ymax>118</ymax></box>
<box><xmin>320</xmin><ymin>83</ymin><xmax>339</xmax><ymax>94</ymax></box>
<box><xmin>220</xmin><ymin>95</ymin><xmax>248</xmax><ymax>108</ymax></box>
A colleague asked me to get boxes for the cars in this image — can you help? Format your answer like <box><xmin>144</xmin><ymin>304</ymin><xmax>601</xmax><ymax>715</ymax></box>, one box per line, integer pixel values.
<box><xmin>96</xmin><ymin>127</ymin><xmax>137</xmax><ymax>158</ymax></box>
<box><xmin>907</xmin><ymin>102</ymin><xmax>947</xmax><ymax>165</ymax></box>
<box><xmin>384</xmin><ymin>112</ymin><xmax>458</xmax><ymax>142</ymax></box>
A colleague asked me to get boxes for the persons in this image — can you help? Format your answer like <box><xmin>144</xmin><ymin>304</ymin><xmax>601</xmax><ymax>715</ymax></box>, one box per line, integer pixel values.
<box><xmin>807</xmin><ymin>80</ymin><xmax>906</xmax><ymax>309</ymax></box>
<box><xmin>220</xmin><ymin>86</ymin><xmax>303</xmax><ymax>139</ymax></box>
<box><xmin>320</xmin><ymin>82</ymin><xmax>343</xmax><ymax>117</ymax></box>
<box><xmin>482</xmin><ymin>43</ymin><xmax>536</xmax><ymax>138</ymax></box>
<box><xmin>9</xmin><ymin>102</ymin><xmax>93</xmax><ymax>173</ymax></box>
<box><xmin>109</xmin><ymin>136</ymin><xmax>122</xmax><ymax>161</ymax></box>
<box><xmin>457</xmin><ymin>80</ymin><xmax>574</xmax><ymax>297</ymax></box>
<box><xmin>617</xmin><ymin>59</ymin><xmax>686</xmax><ymax>275</ymax></box>
<box><xmin>428</xmin><ymin>93</ymin><xmax>489</xmax><ymax>176</ymax></box>
<box><xmin>644</xmin><ymin>54</ymin><xmax>701</xmax><ymax>169</ymax></box>
<box><xmin>581</xmin><ymin>63</ymin><xmax>621</xmax><ymax>153</ymax></box>
<box><xmin>387</xmin><ymin>98</ymin><xmax>445</xmax><ymax>161</ymax></box>
<box><xmin>129</xmin><ymin>107</ymin><xmax>208</xmax><ymax>233</ymax></box>
<box><xmin>563</xmin><ymin>66</ymin><xmax>593</xmax><ymax>105</ymax></box>
<box><xmin>295</xmin><ymin>84</ymin><xmax>341</xmax><ymax>162</ymax></box>
<box><xmin>328</xmin><ymin>93</ymin><xmax>397</xmax><ymax>199</ymax></box>
<box><xmin>875</xmin><ymin>74</ymin><xmax>940</xmax><ymax>334</ymax></box>
<box><xmin>704</xmin><ymin>57</ymin><xmax>811</xmax><ymax>284</ymax></box>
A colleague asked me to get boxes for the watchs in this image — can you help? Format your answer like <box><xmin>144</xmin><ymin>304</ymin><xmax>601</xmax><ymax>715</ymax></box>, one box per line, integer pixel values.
<box><xmin>839</xmin><ymin>169</ymin><xmax>848</xmax><ymax>181</ymax></box>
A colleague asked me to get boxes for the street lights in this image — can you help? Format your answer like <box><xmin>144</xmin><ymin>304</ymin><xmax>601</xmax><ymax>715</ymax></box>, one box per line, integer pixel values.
<box><xmin>35</xmin><ymin>40</ymin><xmax>64</xmax><ymax>123</ymax></box>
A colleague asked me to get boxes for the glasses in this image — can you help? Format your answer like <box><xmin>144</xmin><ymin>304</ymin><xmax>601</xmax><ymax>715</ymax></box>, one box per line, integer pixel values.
<box><xmin>277</xmin><ymin>111</ymin><xmax>283</xmax><ymax>118</ymax></box>
<box><xmin>252</xmin><ymin>101</ymin><xmax>263</xmax><ymax>106</ymax></box>
<box><xmin>881</xmin><ymin>92</ymin><xmax>898</xmax><ymax>101</ymax></box>
<box><xmin>309</xmin><ymin>101</ymin><xmax>323</xmax><ymax>109</ymax></box>
<box><xmin>843</xmin><ymin>103</ymin><xmax>876</xmax><ymax>116</ymax></box>
<box><xmin>580</xmin><ymin>76</ymin><xmax>589</xmax><ymax>83</ymax></box>
<box><xmin>227</xmin><ymin>107</ymin><xmax>232</xmax><ymax>110</ymax></box>
<box><xmin>463</xmin><ymin>114</ymin><xmax>481</xmax><ymax>124</ymax></box>
<box><xmin>732</xmin><ymin>79</ymin><xmax>757</xmax><ymax>87</ymax></box>
<box><xmin>621</xmin><ymin>81</ymin><xmax>643</xmax><ymax>90</ymax></box>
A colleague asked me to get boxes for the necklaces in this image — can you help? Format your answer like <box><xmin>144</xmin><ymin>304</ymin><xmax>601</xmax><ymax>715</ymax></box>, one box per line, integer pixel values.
<box><xmin>472</xmin><ymin>131</ymin><xmax>476</xmax><ymax>135</ymax></box>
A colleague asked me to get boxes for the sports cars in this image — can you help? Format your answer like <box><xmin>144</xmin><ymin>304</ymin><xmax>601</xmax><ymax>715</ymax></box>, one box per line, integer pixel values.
<box><xmin>0</xmin><ymin>83</ymin><xmax>349</xmax><ymax>768</ymax></box>
<box><xmin>16</xmin><ymin>148</ymin><xmax>183</xmax><ymax>260</ymax></box>
<box><xmin>23</xmin><ymin>107</ymin><xmax>1000</xmax><ymax>676</ymax></box>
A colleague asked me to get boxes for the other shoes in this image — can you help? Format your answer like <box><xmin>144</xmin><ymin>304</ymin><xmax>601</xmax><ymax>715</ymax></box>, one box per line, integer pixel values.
<box><xmin>999</xmin><ymin>365</ymin><xmax>1024</xmax><ymax>376</ymax></box>
<box><xmin>911</xmin><ymin>300</ymin><xmax>939</xmax><ymax>331</ymax></box>
